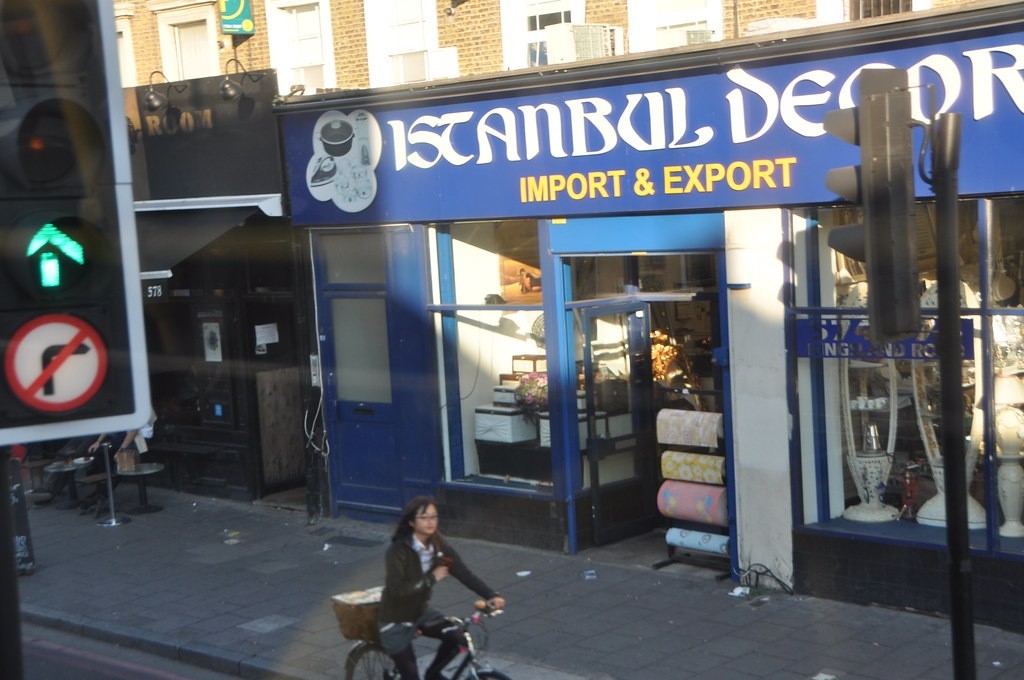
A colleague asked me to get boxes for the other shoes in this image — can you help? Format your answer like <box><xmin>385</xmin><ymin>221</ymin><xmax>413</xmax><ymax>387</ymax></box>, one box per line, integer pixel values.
<box><xmin>27</xmin><ymin>488</ymin><xmax>54</xmax><ymax>509</ymax></box>
<box><xmin>80</xmin><ymin>494</ymin><xmax>106</xmax><ymax>510</ymax></box>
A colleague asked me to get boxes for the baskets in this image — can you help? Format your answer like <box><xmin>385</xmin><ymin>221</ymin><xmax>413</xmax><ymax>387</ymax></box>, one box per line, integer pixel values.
<box><xmin>331</xmin><ymin>585</ymin><xmax>387</xmax><ymax>645</ymax></box>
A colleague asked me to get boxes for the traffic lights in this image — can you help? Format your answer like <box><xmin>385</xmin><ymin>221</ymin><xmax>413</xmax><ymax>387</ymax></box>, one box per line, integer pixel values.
<box><xmin>0</xmin><ymin>2</ymin><xmax>124</xmax><ymax>313</ymax></box>
<box><xmin>827</xmin><ymin>67</ymin><xmax>922</xmax><ymax>329</ymax></box>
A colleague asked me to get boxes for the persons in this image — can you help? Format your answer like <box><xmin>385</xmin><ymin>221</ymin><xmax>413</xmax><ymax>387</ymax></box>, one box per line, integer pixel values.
<box><xmin>44</xmin><ymin>409</ymin><xmax>156</xmax><ymax>519</ymax></box>
<box><xmin>376</xmin><ymin>497</ymin><xmax>505</xmax><ymax>680</ymax></box>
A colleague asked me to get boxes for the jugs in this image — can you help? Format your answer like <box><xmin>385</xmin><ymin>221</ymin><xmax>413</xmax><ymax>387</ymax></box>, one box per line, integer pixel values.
<box><xmin>861</xmin><ymin>422</ymin><xmax>881</xmax><ymax>454</ymax></box>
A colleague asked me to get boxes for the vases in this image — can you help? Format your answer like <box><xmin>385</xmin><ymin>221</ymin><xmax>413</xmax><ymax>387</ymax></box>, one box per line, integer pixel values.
<box><xmin>838</xmin><ymin>279</ymin><xmax>1024</xmax><ymax>538</ymax></box>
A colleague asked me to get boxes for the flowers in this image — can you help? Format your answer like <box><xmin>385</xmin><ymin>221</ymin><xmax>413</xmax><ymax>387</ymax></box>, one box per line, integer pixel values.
<box><xmin>515</xmin><ymin>372</ymin><xmax>549</xmax><ymax>407</ymax></box>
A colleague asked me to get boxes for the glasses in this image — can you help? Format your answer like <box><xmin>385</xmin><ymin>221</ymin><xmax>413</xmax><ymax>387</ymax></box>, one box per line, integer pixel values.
<box><xmin>415</xmin><ymin>513</ymin><xmax>440</xmax><ymax>523</ymax></box>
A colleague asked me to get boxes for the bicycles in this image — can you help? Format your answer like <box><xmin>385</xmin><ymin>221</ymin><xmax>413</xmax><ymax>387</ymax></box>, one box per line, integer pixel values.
<box><xmin>330</xmin><ymin>588</ymin><xmax>511</xmax><ymax>680</ymax></box>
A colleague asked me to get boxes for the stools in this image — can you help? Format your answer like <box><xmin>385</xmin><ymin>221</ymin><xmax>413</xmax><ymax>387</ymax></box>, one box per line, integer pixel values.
<box><xmin>21</xmin><ymin>456</ymin><xmax>165</xmax><ymax>518</ymax></box>
